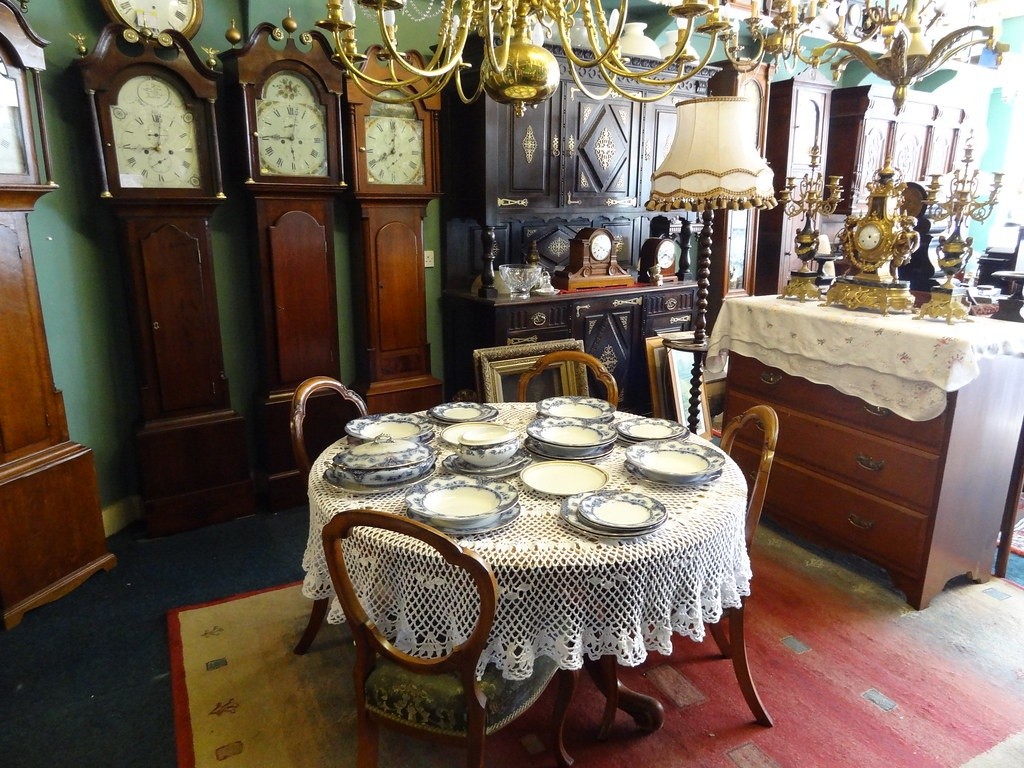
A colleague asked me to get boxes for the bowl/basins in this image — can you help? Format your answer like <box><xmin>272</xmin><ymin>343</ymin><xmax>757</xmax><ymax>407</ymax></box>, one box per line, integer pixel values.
<box><xmin>324</xmin><ymin>435</ymin><xmax>441</xmax><ymax>485</ymax></box>
<box><xmin>458</xmin><ymin>424</ymin><xmax>519</xmax><ymax>467</ymax></box>
<box><xmin>405</xmin><ymin>475</ymin><xmax>519</xmax><ymax>524</ymax></box>
<box><xmin>626</xmin><ymin>439</ymin><xmax>725</xmax><ymax>484</ymax></box>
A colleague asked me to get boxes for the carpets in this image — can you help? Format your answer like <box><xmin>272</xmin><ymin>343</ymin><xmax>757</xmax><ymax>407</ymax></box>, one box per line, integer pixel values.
<box><xmin>165</xmin><ymin>517</ymin><xmax>1024</xmax><ymax>767</ymax></box>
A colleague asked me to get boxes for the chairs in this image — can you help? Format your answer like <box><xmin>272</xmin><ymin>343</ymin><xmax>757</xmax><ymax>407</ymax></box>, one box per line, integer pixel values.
<box><xmin>291</xmin><ymin>376</ymin><xmax>778</xmax><ymax>767</ymax></box>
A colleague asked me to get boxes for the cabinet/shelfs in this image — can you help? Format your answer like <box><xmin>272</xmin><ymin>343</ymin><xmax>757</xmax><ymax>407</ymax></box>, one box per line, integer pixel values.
<box><xmin>705</xmin><ymin>58</ymin><xmax>966</xmax><ymax>337</ymax></box>
<box><xmin>429</xmin><ymin>29</ymin><xmax>722</xmax><ymax>418</ymax></box>
<box><xmin>704</xmin><ymin>293</ymin><xmax>1024</xmax><ymax>612</ymax></box>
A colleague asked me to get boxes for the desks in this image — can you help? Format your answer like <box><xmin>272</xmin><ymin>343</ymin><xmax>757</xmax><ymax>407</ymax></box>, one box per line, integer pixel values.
<box><xmin>301</xmin><ymin>400</ymin><xmax>754</xmax><ymax>744</ymax></box>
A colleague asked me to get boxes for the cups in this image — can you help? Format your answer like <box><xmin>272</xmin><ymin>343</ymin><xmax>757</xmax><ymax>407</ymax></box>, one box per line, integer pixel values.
<box><xmin>499</xmin><ymin>265</ymin><xmax>541</xmax><ymax>299</ymax></box>
<box><xmin>992</xmin><ymin>271</ymin><xmax>1024</xmax><ymax>299</ymax></box>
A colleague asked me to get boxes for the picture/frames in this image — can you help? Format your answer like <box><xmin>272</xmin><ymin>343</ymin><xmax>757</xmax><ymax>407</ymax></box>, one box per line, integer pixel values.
<box><xmin>646</xmin><ymin>331</ymin><xmax>714</xmax><ymax>441</ymax></box>
<box><xmin>473</xmin><ymin>338</ymin><xmax>590</xmax><ymax>404</ymax></box>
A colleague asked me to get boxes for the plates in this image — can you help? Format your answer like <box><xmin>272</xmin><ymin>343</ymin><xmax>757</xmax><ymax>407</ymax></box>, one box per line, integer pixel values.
<box><xmin>526</xmin><ymin>417</ymin><xmax>618</xmax><ymax>450</ymax></box>
<box><xmin>609</xmin><ymin>418</ymin><xmax>691</xmax><ymax>446</ymax></box>
<box><xmin>406</xmin><ymin>502</ymin><xmax>521</xmax><ymax>535</ymax></box>
<box><xmin>322</xmin><ymin>464</ymin><xmax>436</xmax><ymax>494</ymax></box>
<box><xmin>520</xmin><ymin>461</ymin><xmax>609</xmax><ymax>495</ymax></box>
<box><xmin>346</xmin><ymin>431</ymin><xmax>435</xmax><ymax>444</ymax></box>
<box><xmin>560</xmin><ymin>490</ymin><xmax>668</xmax><ymax>539</ymax></box>
<box><xmin>536</xmin><ymin>396</ymin><xmax>616</xmax><ymax>424</ymax></box>
<box><xmin>624</xmin><ymin>459</ymin><xmax>726</xmax><ymax>485</ymax></box>
<box><xmin>535</xmin><ymin>289</ymin><xmax>560</xmax><ymax>294</ymax></box>
<box><xmin>345</xmin><ymin>412</ymin><xmax>433</xmax><ymax>439</ymax></box>
<box><xmin>523</xmin><ymin>436</ymin><xmax>615</xmax><ymax>461</ymax></box>
<box><xmin>443</xmin><ymin>449</ymin><xmax>532</xmax><ymax>478</ymax></box>
<box><xmin>427</xmin><ymin>401</ymin><xmax>499</xmax><ymax>425</ymax></box>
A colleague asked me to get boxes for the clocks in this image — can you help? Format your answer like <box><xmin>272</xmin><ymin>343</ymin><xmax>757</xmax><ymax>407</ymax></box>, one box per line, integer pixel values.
<box><xmin>0</xmin><ymin>1</ymin><xmax>450</xmax><ymax>629</ymax></box>
<box><xmin>554</xmin><ymin>227</ymin><xmax>678</xmax><ymax>290</ymax></box>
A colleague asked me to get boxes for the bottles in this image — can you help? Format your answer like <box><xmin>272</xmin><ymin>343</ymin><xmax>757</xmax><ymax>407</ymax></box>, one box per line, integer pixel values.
<box><xmin>541</xmin><ymin>271</ymin><xmax>554</xmax><ymax>291</ymax></box>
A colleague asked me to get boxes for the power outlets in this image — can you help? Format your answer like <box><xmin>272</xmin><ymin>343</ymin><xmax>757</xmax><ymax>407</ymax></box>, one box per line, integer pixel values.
<box><xmin>424</xmin><ymin>250</ymin><xmax>435</xmax><ymax>268</ymax></box>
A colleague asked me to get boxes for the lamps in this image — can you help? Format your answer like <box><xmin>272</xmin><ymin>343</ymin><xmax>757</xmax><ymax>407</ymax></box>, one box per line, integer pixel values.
<box><xmin>642</xmin><ymin>95</ymin><xmax>777</xmax><ymax>438</ymax></box>
<box><xmin>316</xmin><ymin>0</ymin><xmax>1002</xmax><ymax>118</ymax></box>
